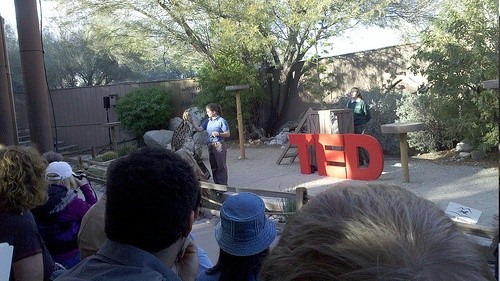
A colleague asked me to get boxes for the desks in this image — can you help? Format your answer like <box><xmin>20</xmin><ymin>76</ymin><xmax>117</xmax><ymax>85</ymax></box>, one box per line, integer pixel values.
<box><xmin>381</xmin><ymin>122</ymin><xmax>425</xmax><ymax>182</ymax></box>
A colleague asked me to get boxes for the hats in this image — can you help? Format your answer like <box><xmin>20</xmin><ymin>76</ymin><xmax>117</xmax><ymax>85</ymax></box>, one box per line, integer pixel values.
<box><xmin>214</xmin><ymin>192</ymin><xmax>276</xmax><ymax>256</ymax></box>
<box><xmin>45</xmin><ymin>161</ymin><xmax>75</xmax><ymax>181</ymax></box>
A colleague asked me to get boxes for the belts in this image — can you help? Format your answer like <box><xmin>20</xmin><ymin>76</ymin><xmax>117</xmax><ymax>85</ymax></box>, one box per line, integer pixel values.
<box><xmin>209</xmin><ymin>140</ymin><xmax>223</xmax><ymax>145</ymax></box>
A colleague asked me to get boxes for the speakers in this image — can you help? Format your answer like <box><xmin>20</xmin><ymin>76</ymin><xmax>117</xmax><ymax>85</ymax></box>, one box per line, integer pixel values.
<box><xmin>104</xmin><ymin>97</ymin><xmax>110</xmax><ymax>108</ymax></box>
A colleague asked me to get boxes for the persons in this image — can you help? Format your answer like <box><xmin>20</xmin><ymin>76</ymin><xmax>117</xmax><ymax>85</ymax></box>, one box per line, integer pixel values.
<box><xmin>0</xmin><ymin>146</ymin><xmax>212</xmax><ymax>281</ymax></box>
<box><xmin>187</xmin><ymin>103</ymin><xmax>231</xmax><ymax>186</ymax></box>
<box><xmin>347</xmin><ymin>88</ymin><xmax>371</xmax><ymax>166</ymax></box>
<box><xmin>263</xmin><ymin>179</ymin><xmax>497</xmax><ymax>281</ymax></box>
<box><xmin>195</xmin><ymin>192</ymin><xmax>276</xmax><ymax>281</ymax></box>
